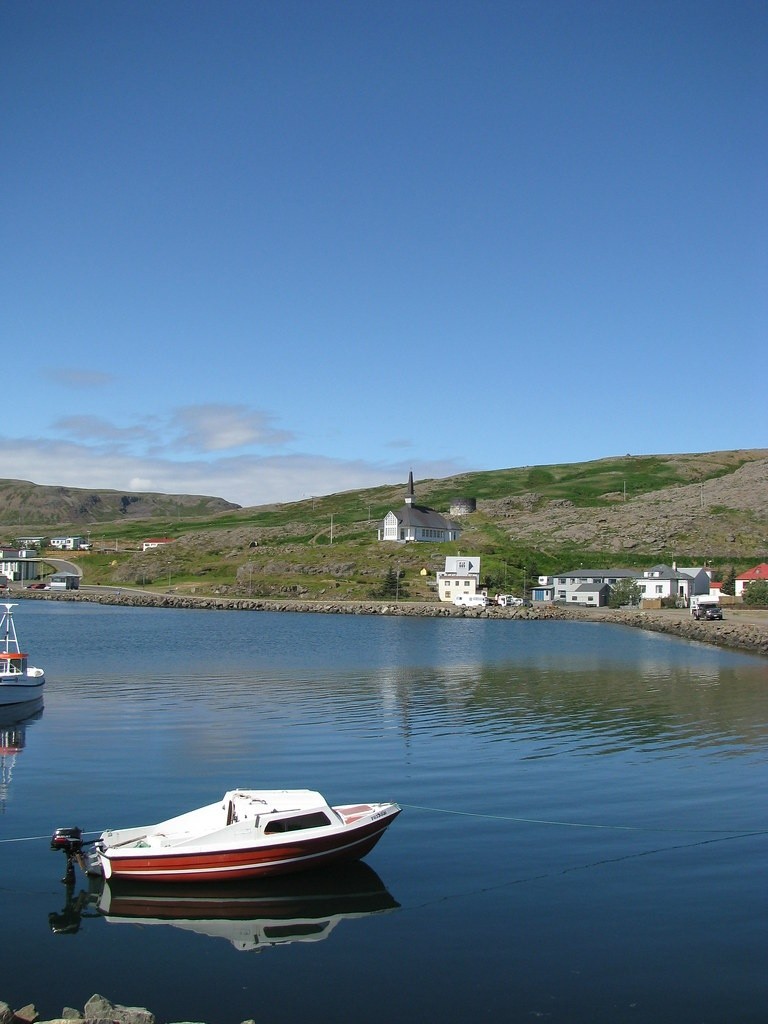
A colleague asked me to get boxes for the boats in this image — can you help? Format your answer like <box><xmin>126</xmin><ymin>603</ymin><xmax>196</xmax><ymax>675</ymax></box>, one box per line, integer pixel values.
<box><xmin>0</xmin><ymin>602</ymin><xmax>45</xmax><ymax>704</ymax></box>
<box><xmin>50</xmin><ymin>788</ymin><xmax>404</xmax><ymax>881</ymax></box>
<box><xmin>0</xmin><ymin>695</ymin><xmax>45</xmax><ymax>802</ymax></box>
<box><xmin>51</xmin><ymin>859</ymin><xmax>403</xmax><ymax>954</ymax></box>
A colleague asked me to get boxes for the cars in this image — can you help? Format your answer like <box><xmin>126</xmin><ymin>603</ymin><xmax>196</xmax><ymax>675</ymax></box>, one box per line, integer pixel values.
<box><xmin>491</xmin><ymin>591</ymin><xmax>542</xmax><ymax>609</ymax></box>
<box><xmin>0</xmin><ymin>579</ymin><xmax>53</xmax><ymax>591</ymax></box>
<box><xmin>704</xmin><ymin>607</ymin><xmax>724</xmax><ymax>621</ymax></box>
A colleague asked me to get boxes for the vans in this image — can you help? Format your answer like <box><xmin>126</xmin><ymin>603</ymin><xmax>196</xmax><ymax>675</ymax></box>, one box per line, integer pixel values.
<box><xmin>452</xmin><ymin>594</ymin><xmax>489</xmax><ymax>606</ymax></box>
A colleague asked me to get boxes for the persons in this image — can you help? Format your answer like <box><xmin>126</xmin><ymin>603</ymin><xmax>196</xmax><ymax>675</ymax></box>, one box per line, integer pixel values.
<box><xmin>493</xmin><ymin>593</ymin><xmax>498</xmax><ymax>606</ymax></box>
